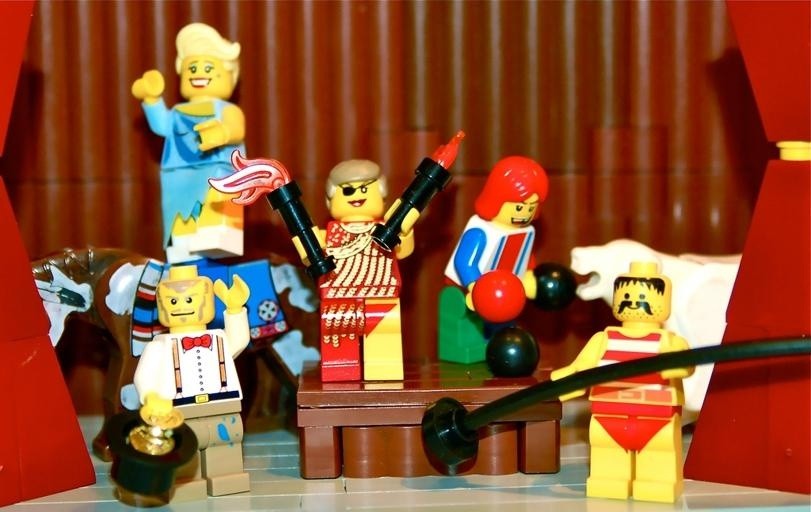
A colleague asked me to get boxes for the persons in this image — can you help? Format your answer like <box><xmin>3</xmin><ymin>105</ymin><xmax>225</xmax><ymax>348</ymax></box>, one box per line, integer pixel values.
<box><xmin>33</xmin><ymin>15</ymin><xmax>699</xmax><ymax>505</ymax></box>
